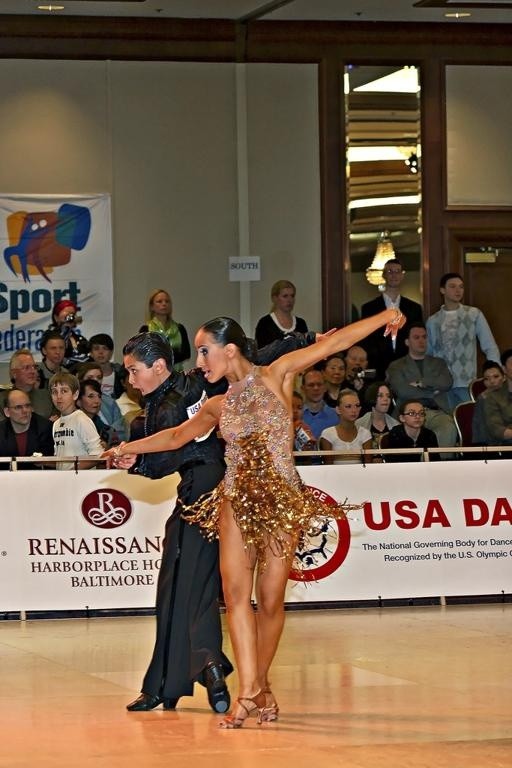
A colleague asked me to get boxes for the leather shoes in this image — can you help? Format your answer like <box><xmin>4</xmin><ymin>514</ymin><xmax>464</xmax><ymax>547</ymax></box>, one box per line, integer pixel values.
<box><xmin>203</xmin><ymin>662</ymin><xmax>231</xmax><ymax>713</ymax></box>
<box><xmin>125</xmin><ymin>686</ymin><xmax>182</xmax><ymax>712</ymax></box>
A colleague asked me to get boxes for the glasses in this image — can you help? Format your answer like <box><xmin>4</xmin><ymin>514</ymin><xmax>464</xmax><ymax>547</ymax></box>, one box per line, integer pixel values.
<box><xmin>402</xmin><ymin>411</ymin><xmax>426</xmax><ymax>417</ymax></box>
<box><xmin>11</xmin><ymin>363</ymin><xmax>38</xmax><ymax>370</ymax></box>
<box><xmin>383</xmin><ymin>268</ymin><xmax>401</xmax><ymax>274</ymax></box>
<box><xmin>5</xmin><ymin>403</ymin><xmax>32</xmax><ymax>410</ymax></box>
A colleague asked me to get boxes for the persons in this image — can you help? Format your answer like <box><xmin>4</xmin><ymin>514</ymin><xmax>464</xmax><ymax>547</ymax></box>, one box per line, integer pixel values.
<box><xmin>292</xmin><ymin>391</ymin><xmax>314</xmax><ymax>467</ymax></box>
<box><xmin>385</xmin><ymin>323</ymin><xmax>453</xmax><ymax>410</ymax></box>
<box><xmin>0</xmin><ymin>348</ymin><xmax>60</xmax><ymax>422</ymax></box>
<box><xmin>34</xmin><ymin>333</ymin><xmax>71</xmax><ymax>390</ymax></box>
<box><xmin>424</xmin><ymin>272</ymin><xmax>502</xmax><ymax>408</ymax></box>
<box><xmin>42</xmin><ymin>300</ymin><xmax>93</xmax><ymax>374</ymax></box>
<box><xmin>114</xmin><ymin>363</ymin><xmax>146</xmax><ymax>424</ymax></box>
<box><xmin>34</xmin><ymin>372</ymin><xmax>104</xmax><ymax>470</ymax></box>
<box><xmin>138</xmin><ymin>288</ymin><xmax>191</xmax><ymax>374</ymax></box>
<box><xmin>0</xmin><ymin>388</ymin><xmax>56</xmax><ymax>471</ymax></box>
<box><xmin>361</xmin><ymin>259</ymin><xmax>423</xmax><ymax>377</ymax></box>
<box><xmin>482</xmin><ymin>358</ymin><xmax>506</xmax><ymax>390</ymax></box>
<box><xmin>76</xmin><ymin>379</ymin><xmax>119</xmax><ymax>452</ymax></box>
<box><xmin>88</xmin><ymin>333</ymin><xmax>123</xmax><ymax>400</ymax></box>
<box><xmin>344</xmin><ymin>345</ymin><xmax>378</xmax><ymax>419</ymax></box>
<box><xmin>384</xmin><ymin>399</ymin><xmax>441</xmax><ymax>463</ymax></box>
<box><xmin>112</xmin><ymin>325</ymin><xmax>338</xmax><ymax>714</ymax></box>
<box><xmin>472</xmin><ymin>349</ymin><xmax>512</xmax><ymax>460</ymax></box>
<box><xmin>354</xmin><ymin>381</ymin><xmax>400</xmax><ymax>463</ymax></box>
<box><xmin>98</xmin><ymin>305</ymin><xmax>407</xmax><ymax>729</ymax></box>
<box><xmin>254</xmin><ymin>280</ymin><xmax>308</xmax><ymax>347</ymax></box>
<box><xmin>321</xmin><ymin>356</ymin><xmax>347</xmax><ymax>408</ymax></box>
<box><xmin>300</xmin><ymin>369</ymin><xmax>339</xmax><ymax>439</ymax></box>
<box><xmin>319</xmin><ymin>388</ymin><xmax>373</xmax><ymax>465</ymax></box>
<box><xmin>76</xmin><ymin>363</ymin><xmax>126</xmax><ymax>443</ymax></box>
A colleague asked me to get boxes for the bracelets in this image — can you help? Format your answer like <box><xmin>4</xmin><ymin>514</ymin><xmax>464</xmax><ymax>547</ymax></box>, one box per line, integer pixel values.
<box><xmin>113</xmin><ymin>445</ymin><xmax>121</xmax><ymax>459</ymax></box>
<box><xmin>415</xmin><ymin>380</ymin><xmax>420</xmax><ymax>388</ymax></box>
<box><xmin>390</xmin><ymin>307</ymin><xmax>402</xmax><ymax>325</ymax></box>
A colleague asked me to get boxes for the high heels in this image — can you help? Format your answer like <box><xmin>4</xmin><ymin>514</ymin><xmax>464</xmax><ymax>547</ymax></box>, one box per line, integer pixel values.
<box><xmin>217</xmin><ymin>689</ymin><xmax>266</xmax><ymax>730</ymax></box>
<box><xmin>258</xmin><ymin>685</ymin><xmax>279</xmax><ymax>724</ymax></box>
